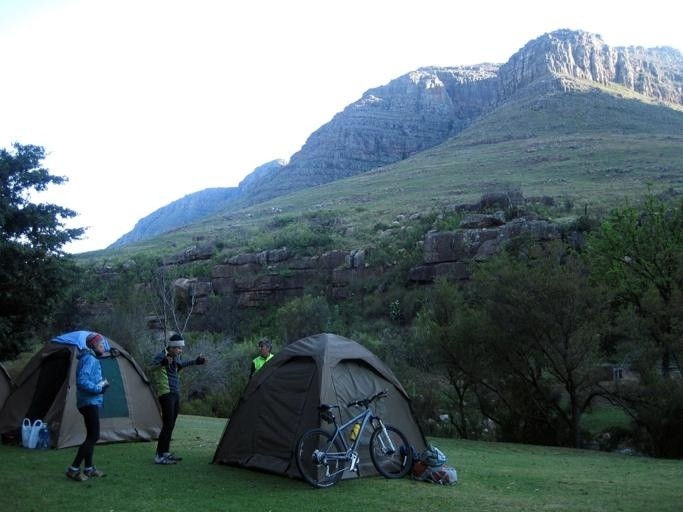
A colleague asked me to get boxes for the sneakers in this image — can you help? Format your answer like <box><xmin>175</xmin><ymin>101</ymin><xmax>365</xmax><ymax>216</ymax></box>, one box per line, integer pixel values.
<box><xmin>155</xmin><ymin>458</ymin><xmax>176</xmax><ymax>465</ymax></box>
<box><xmin>166</xmin><ymin>452</ymin><xmax>182</xmax><ymax>460</ymax></box>
<box><xmin>85</xmin><ymin>465</ymin><xmax>108</xmax><ymax>477</ymax></box>
<box><xmin>65</xmin><ymin>466</ymin><xmax>89</xmax><ymax>481</ymax></box>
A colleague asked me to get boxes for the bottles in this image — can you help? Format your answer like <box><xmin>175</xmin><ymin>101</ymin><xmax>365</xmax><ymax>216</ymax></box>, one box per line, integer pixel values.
<box><xmin>349</xmin><ymin>420</ymin><xmax>362</xmax><ymax>442</ymax></box>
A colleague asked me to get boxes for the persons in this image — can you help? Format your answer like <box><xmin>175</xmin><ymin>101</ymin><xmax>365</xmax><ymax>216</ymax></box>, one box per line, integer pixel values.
<box><xmin>141</xmin><ymin>334</ymin><xmax>208</xmax><ymax>465</ymax></box>
<box><xmin>248</xmin><ymin>337</ymin><xmax>272</xmax><ymax>373</ymax></box>
<box><xmin>64</xmin><ymin>331</ymin><xmax>109</xmax><ymax>481</ymax></box>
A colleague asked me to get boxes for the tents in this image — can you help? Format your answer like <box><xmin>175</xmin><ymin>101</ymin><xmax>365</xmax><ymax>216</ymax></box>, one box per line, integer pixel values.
<box><xmin>0</xmin><ymin>330</ymin><xmax>169</xmax><ymax>449</ymax></box>
<box><xmin>211</xmin><ymin>332</ymin><xmax>429</xmax><ymax>481</ymax></box>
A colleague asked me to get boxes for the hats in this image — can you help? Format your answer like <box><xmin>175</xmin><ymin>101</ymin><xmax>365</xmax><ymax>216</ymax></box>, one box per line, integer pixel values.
<box><xmin>168</xmin><ymin>334</ymin><xmax>185</xmax><ymax>346</ymax></box>
<box><xmin>87</xmin><ymin>334</ymin><xmax>104</xmax><ymax>349</ymax></box>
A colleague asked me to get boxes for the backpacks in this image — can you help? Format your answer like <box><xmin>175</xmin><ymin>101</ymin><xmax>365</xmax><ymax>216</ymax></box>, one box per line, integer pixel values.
<box><xmin>413</xmin><ymin>461</ymin><xmax>458</xmax><ymax>487</ymax></box>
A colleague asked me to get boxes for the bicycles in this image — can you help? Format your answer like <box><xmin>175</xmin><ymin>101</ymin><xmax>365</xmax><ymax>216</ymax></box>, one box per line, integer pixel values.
<box><xmin>295</xmin><ymin>390</ymin><xmax>414</xmax><ymax>486</ymax></box>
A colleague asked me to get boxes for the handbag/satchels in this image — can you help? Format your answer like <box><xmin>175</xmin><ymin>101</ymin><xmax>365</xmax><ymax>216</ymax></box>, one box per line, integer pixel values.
<box><xmin>421</xmin><ymin>443</ymin><xmax>447</xmax><ymax>466</ymax></box>
<box><xmin>22</xmin><ymin>419</ymin><xmax>53</xmax><ymax>450</ymax></box>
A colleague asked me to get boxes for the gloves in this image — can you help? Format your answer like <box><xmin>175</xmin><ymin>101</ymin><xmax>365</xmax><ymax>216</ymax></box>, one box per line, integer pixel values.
<box><xmin>101</xmin><ymin>387</ymin><xmax>105</xmax><ymax>395</ymax></box>
